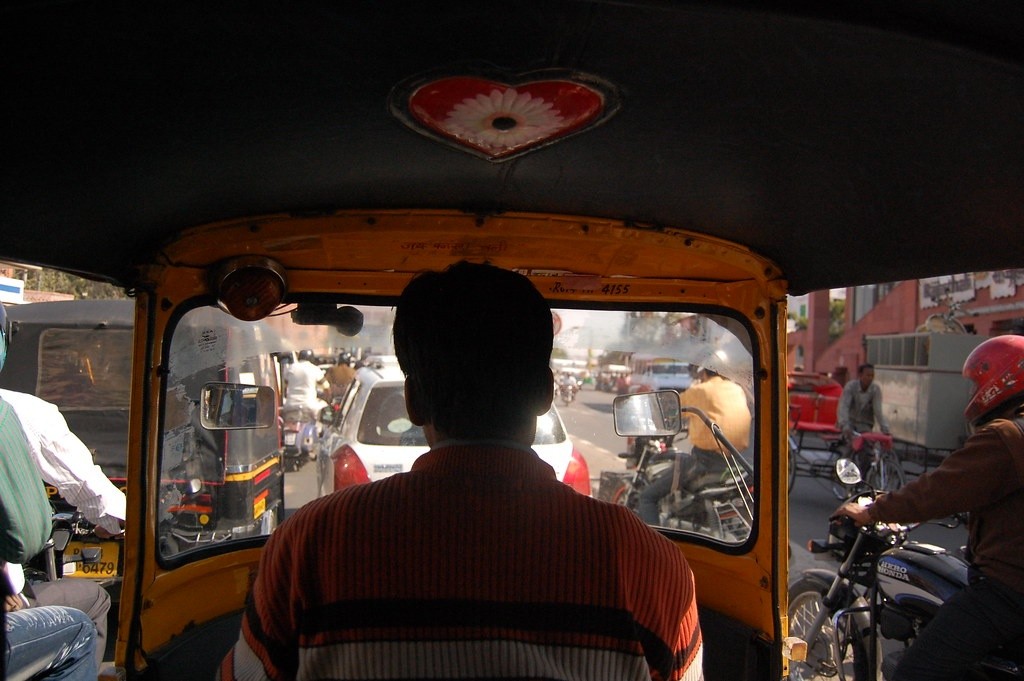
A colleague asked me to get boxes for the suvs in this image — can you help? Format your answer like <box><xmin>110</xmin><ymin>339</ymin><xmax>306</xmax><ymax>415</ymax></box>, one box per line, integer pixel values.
<box><xmin>314</xmin><ymin>355</ymin><xmax>591</xmax><ymax>517</ymax></box>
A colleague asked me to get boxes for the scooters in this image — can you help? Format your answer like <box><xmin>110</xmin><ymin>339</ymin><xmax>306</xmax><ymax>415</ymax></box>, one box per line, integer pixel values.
<box><xmin>559</xmin><ymin>384</ymin><xmax>576</xmax><ymax>406</ymax></box>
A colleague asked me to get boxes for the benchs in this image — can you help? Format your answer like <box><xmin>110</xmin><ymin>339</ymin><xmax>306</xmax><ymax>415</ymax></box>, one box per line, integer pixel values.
<box><xmin>788</xmin><ymin>383</ymin><xmax>843</xmax><ymax>438</ymax></box>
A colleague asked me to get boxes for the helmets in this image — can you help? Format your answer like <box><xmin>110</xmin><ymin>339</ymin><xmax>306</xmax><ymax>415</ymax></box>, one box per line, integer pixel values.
<box><xmin>961</xmin><ymin>334</ymin><xmax>1024</xmax><ymax>427</ymax></box>
<box><xmin>338</xmin><ymin>353</ymin><xmax>350</xmax><ymax>366</ymax></box>
<box><xmin>298</xmin><ymin>349</ymin><xmax>313</xmax><ymax>360</ymax></box>
<box><xmin>690</xmin><ymin>344</ymin><xmax>731</xmax><ymax>380</ymax></box>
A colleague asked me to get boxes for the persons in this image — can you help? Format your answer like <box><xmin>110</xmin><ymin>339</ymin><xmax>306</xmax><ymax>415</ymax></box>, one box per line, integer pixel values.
<box><xmin>553</xmin><ymin>369</ymin><xmax>579</xmax><ymax>399</ymax></box>
<box><xmin>638</xmin><ymin>346</ymin><xmax>751</xmax><ymax>526</ymax></box>
<box><xmin>219</xmin><ymin>260</ymin><xmax>704</xmax><ymax>680</ymax></box>
<box><xmin>595</xmin><ymin>370</ymin><xmax>631</xmax><ymax>392</ymax></box>
<box><xmin>280</xmin><ymin>348</ymin><xmax>370</xmax><ymax>462</ymax></box>
<box><xmin>1</xmin><ymin>304</ymin><xmax>127</xmax><ymax>681</ymax></box>
<box><xmin>827</xmin><ymin>333</ymin><xmax>1024</xmax><ymax>681</ymax></box>
<box><xmin>838</xmin><ymin>364</ymin><xmax>894</xmax><ymax>483</ymax></box>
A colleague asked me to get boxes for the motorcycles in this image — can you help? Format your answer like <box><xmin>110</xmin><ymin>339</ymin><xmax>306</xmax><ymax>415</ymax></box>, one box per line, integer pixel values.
<box><xmin>608</xmin><ymin>434</ymin><xmax>794</xmax><ymax>561</ymax></box>
<box><xmin>26</xmin><ymin>500</ymin><xmax>110</xmax><ymax>590</ymax></box>
<box><xmin>282</xmin><ymin>404</ymin><xmax>320</xmax><ymax>473</ymax></box>
<box><xmin>2</xmin><ymin>300</ymin><xmax>287</xmax><ymax>580</ymax></box>
<box><xmin>785</xmin><ymin>459</ymin><xmax>1024</xmax><ymax>681</ymax></box>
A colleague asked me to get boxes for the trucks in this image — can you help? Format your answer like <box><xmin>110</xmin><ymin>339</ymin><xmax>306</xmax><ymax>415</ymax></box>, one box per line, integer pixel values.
<box><xmin>628</xmin><ymin>361</ymin><xmax>692</xmax><ymax>402</ymax></box>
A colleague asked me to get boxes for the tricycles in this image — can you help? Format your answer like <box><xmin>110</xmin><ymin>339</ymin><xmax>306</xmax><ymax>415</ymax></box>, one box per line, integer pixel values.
<box><xmin>788</xmin><ymin>371</ymin><xmax>907</xmax><ymax>492</ymax></box>
